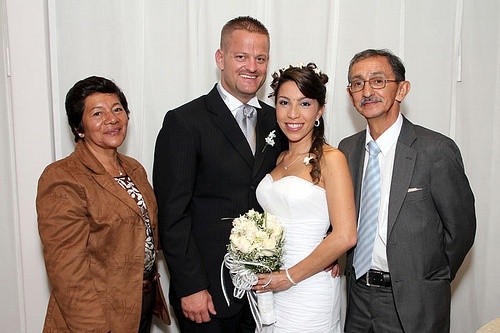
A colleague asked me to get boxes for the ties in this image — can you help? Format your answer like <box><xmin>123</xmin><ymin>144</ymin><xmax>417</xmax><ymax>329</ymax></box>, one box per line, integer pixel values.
<box><xmin>352</xmin><ymin>141</ymin><xmax>381</xmax><ymax>280</ymax></box>
<box><xmin>235</xmin><ymin>105</ymin><xmax>257</xmax><ymax>157</ymax></box>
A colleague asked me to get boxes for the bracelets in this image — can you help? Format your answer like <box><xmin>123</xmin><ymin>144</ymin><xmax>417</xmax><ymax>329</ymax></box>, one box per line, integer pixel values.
<box><xmin>286</xmin><ymin>268</ymin><xmax>297</xmax><ymax>286</ymax></box>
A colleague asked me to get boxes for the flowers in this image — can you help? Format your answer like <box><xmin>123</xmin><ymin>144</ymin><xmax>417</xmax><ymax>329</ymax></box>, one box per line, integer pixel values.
<box><xmin>261</xmin><ymin>129</ymin><xmax>276</xmax><ymax>153</ymax></box>
<box><xmin>221</xmin><ymin>207</ymin><xmax>291</xmax><ymax>326</ymax></box>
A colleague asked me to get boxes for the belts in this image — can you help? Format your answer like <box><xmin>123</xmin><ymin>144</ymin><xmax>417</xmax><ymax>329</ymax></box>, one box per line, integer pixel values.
<box><xmin>360</xmin><ymin>271</ymin><xmax>392</xmax><ymax>288</ymax></box>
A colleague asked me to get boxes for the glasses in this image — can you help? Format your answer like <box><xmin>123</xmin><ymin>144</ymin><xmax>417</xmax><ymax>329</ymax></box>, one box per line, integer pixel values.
<box><xmin>346</xmin><ymin>77</ymin><xmax>400</xmax><ymax>93</ymax></box>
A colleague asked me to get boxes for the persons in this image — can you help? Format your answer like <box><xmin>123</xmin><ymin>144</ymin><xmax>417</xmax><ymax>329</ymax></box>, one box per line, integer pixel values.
<box><xmin>36</xmin><ymin>75</ymin><xmax>171</xmax><ymax>332</ymax></box>
<box><xmin>249</xmin><ymin>63</ymin><xmax>358</xmax><ymax>333</ymax></box>
<box><xmin>153</xmin><ymin>15</ymin><xmax>340</xmax><ymax>333</ymax></box>
<box><xmin>337</xmin><ymin>48</ymin><xmax>477</xmax><ymax>333</ymax></box>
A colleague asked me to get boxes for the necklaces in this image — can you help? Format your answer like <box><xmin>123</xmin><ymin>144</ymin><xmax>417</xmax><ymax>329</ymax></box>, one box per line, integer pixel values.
<box><xmin>118</xmin><ymin>165</ymin><xmax>123</xmax><ymax>176</ymax></box>
<box><xmin>283</xmin><ymin>143</ymin><xmax>310</xmax><ymax>170</ymax></box>
